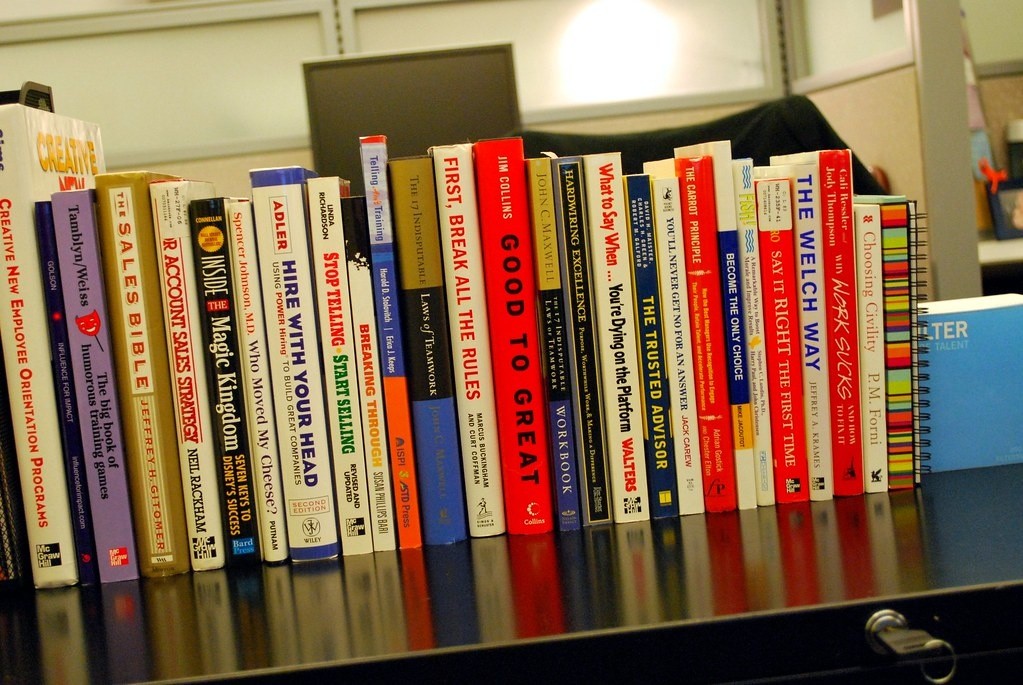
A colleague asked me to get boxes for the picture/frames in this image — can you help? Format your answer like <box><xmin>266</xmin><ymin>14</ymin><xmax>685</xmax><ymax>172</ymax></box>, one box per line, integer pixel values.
<box><xmin>985</xmin><ymin>177</ymin><xmax>1023</xmax><ymax>241</ymax></box>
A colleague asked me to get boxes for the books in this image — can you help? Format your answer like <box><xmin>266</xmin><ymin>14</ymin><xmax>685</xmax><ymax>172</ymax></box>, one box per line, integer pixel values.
<box><xmin>40</xmin><ymin>487</ymin><xmax>926</xmax><ymax>685</ymax></box>
<box><xmin>191</xmin><ymin>196</ymin><xmax>259</xmax><ymax>566</ymax></box>
<box><xmin>880</xmin><ymin>199</ymin><xmax>915</xmax><ymax>490</ymax></box>
<box><xmin>307</xmin><ymin>175</ymin><xmax>374</xmax><ymax>556</ymax></box>
<box><xmin>756</xmin><ymin>178</ymin><xmax>806</xmax><ymax>506</ymax></box>
<box><xmin>919</xmin><ymin>292</ymin><xmax>1023</xmax><ymax>473</ymax></box>
<box><xmin>2</xmin><ymin>103</ymin><xmax>929</xmax><ymax>587</ymax></box>
<box><xmin>474</xmin><ymin>137</ymin><xmax>554</xmax><ymax>536</ymax></box>
